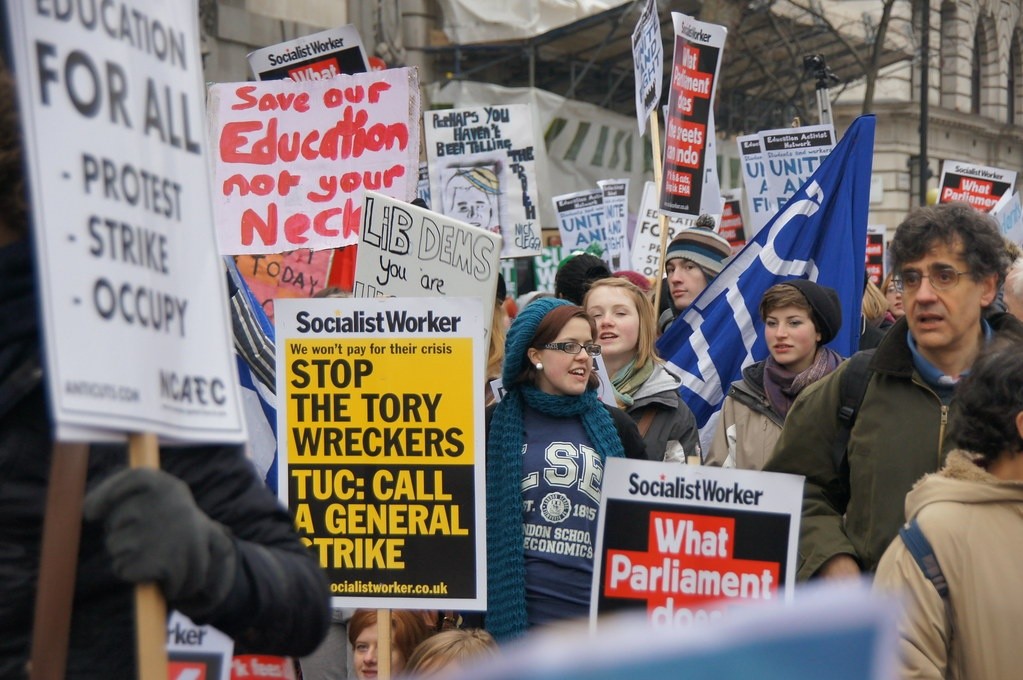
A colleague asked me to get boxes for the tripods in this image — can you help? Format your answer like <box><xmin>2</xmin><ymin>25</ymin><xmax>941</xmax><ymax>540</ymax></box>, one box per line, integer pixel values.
<box><xmin>815</xmin><ymin>66</ymin><xmax>840</xmax><ymax>131</ymax></box>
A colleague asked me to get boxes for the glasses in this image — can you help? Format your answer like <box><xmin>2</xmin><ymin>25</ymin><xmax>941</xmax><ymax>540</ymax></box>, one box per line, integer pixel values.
<box><xmin>541</xmin><ymin>343</ymin><xmax>602</xmax><ymax>357</ymax></box>
<box><xmin>891</xmin><ymin>268</ymin><xmax>973</xmax><ymax>294</ymax></box>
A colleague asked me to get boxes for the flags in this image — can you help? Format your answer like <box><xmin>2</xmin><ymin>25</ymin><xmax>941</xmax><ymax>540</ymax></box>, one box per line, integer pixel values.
<box><xmin>666</xmin><ymin>114</ymin><xmax>874</xmax><ymax>429</ymax></box>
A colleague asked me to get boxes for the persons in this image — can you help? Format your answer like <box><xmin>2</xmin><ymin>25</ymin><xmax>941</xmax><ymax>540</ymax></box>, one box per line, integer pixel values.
<box><xmin>299</xmin><ymin>198</ymin><xmax>1023</xmax><ymax>680</ymax></box>
<box><xmin>0</xmin><ymin>61</ymin><xmax>334</xmax><ymax>680</ymax></box>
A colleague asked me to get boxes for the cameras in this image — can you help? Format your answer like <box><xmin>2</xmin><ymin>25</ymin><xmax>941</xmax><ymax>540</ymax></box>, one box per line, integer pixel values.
<box><xmin>803</xmin><ymin>53</ymin><xmax>827</xmax><ymax>70</ymax></box>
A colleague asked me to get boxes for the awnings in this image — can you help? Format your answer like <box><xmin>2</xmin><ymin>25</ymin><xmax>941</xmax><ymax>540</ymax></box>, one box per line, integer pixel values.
<box><xmin>430</xmin><ymin>0</ymin><xmax>921</xmax><ymax>104</ymax></box>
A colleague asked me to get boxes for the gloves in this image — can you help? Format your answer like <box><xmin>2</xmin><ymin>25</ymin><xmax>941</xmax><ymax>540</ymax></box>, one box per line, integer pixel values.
<box><xmin>85</xmin><ymin>466</ymin><xmax>237</xmax><ymax>624</ymax></box>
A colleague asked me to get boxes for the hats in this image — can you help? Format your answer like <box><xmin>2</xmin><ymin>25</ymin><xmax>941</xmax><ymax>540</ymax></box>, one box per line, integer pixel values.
<box><xmin>502</xmin><ymin>297</ymin><xmax>576</xmax><ymax>391</ymax></box>
<box><xmin>665</xmin><ymin>214</ymin><xmax>732</xmax><ymax>274</ymax></box>
<box><xmin>783</xmin><ymin>279</ymin><xmax>841</xmax><ymax>345</ymax></box>
